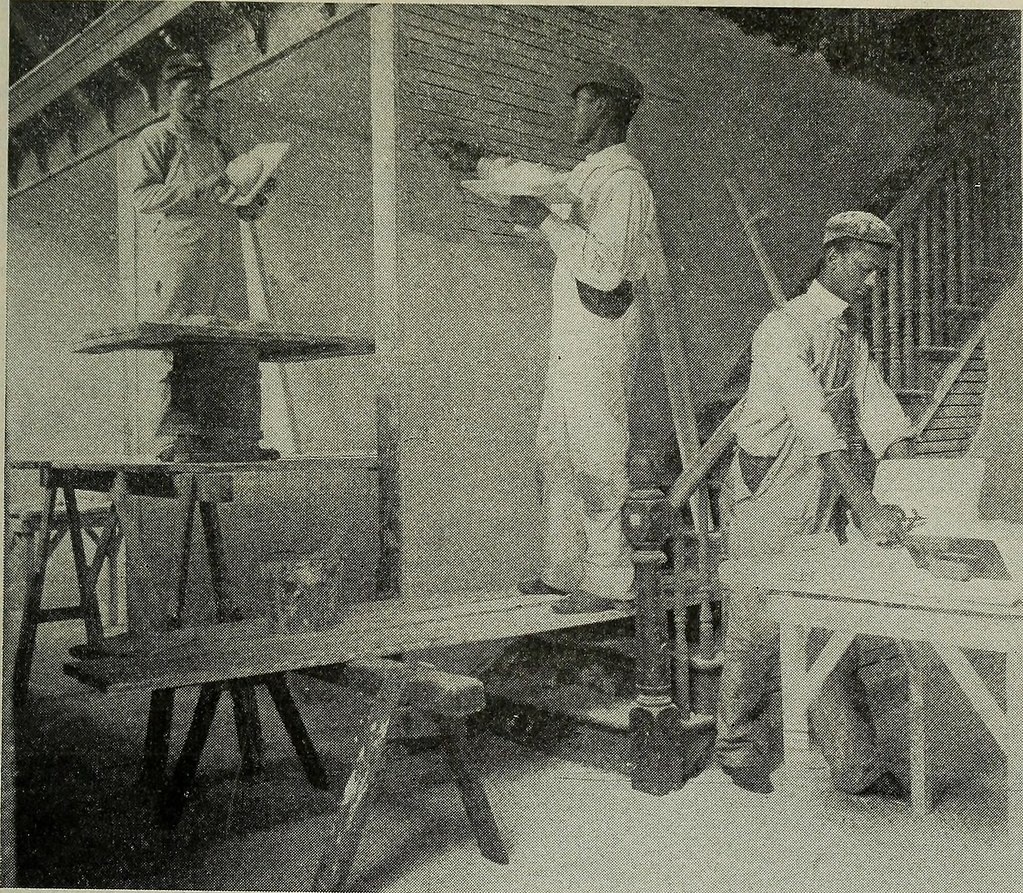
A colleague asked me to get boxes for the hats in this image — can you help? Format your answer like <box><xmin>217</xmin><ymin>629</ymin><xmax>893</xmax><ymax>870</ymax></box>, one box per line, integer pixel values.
<box><xmin>564</xmin><ymin>61</ymin><xmax>643</xmax><ymax>111</ymax></box>
<box><xmin>822</xmin><ymin>211</ymin><xmax>899</xmax><ymax>249</ymax></box>
<box><xmin>161</xmin><ymin>54</ymin><xmax>213</xmax><ymax>81</ymax></box>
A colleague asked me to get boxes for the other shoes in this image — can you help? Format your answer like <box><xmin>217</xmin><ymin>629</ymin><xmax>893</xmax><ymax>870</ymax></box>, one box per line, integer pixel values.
<box><xmin>218</xmin><ymin>441</ymin><xmax>278</xmax><ymax>461</ymax></box>
<box><xmin>863</xmin><ymin>775</ymin><xmax>908</xmax><ymax>797</ymax></box>
<box><xmin>158</xmin><ymin>440</ymin><xmax>218</xmax><ymax>462</ymax></box>
<box><xmin>518</xmin><ymin>576</ymin><xmax>565</xmax><ymax>593</ymax></box>
<box><xmin>721</xmin><ymin>762</ymin><xmax>773</xmax><ymax>794</ymax></box>
<box><xmin>551</xmin><ymin>590</ymin><xmax>631</xmax><ymax>615</ymax></box>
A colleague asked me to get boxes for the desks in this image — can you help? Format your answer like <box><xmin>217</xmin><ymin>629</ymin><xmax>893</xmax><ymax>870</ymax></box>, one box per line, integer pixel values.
<box><xmin>718</xmin><ymin>521</ymin><xmax>1023</xmax><ymax>834</ymax></box>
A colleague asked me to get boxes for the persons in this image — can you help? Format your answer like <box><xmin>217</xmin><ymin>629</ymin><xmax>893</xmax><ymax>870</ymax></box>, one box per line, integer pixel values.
<box><xmin>713</xmin><ymin>210</ymin><xmax>922</xmax><ymax>801</ymax></box>
<box><xmin>430</xmin><ymin>64</ymin><xmax>652</xmax><ymax>614</ymax></box>
<box><xmin>130</xmin><ymin>53</ymin><xmax>281</xmax><ymax>462</ymax></box>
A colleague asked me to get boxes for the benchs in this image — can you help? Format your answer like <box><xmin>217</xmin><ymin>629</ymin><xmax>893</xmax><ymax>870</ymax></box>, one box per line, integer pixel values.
<box><xmin>175</xmin><ymin>625</ymin><xmax>508</xmax><ymax>893</ymax></box>
